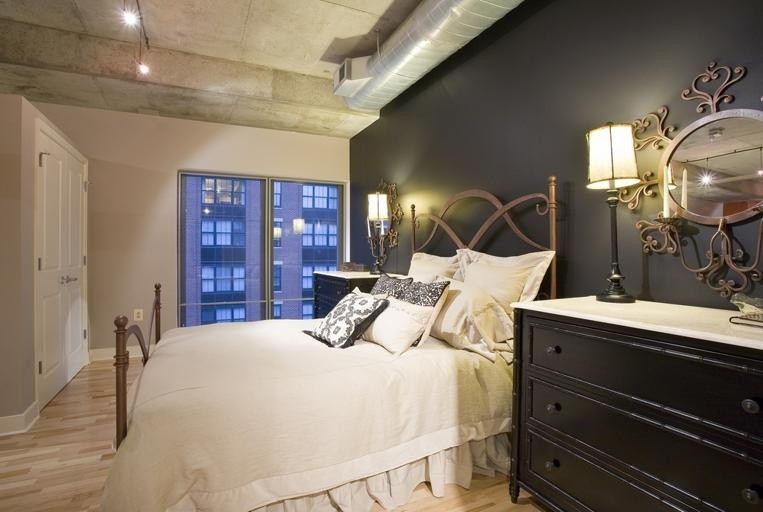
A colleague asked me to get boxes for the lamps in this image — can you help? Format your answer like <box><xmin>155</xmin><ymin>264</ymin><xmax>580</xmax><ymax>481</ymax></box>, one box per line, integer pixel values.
<box><xmin>367</xmin><ymin>193</ymin><xmax>389</xmax><ymax>274</ymax></box>
<box><xmin>585</xmin><ymin>121</ymin><xmax>641</xmax><ymax>303</ymax></box>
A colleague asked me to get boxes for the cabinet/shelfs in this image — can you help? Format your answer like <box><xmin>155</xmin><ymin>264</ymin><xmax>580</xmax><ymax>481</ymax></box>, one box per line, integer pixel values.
<box><xmin>312</xmin><ymin>268</ymin><xmax>405</xmax><ymax>318</ymax></box>
<box><xmin>0</xmin><ymin>93</ymin><xmax>88</xmax><ymax>436</ymax></box>
<box><xmin>509</xmin><ymin>292</ymin><xmax>763</xmax><ymax>512</ymax></box>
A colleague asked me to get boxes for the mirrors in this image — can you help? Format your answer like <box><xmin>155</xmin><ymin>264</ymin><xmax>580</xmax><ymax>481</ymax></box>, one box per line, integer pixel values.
<box><xmin>365</xmin><ymin>176</ymin><xmax>404</xmax><ymax>266</ymax></box>
<box><xmin>616</xmin><ymin>62</ymin><xmax>763</xmax><ymax>300</ymax></box>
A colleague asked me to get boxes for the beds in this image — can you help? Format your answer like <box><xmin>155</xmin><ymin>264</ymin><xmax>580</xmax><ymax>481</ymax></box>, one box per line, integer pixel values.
<box><xmin>95</xmin><ymin>173</ymin><xmax>557</xmax><ymax>512</ymax></box>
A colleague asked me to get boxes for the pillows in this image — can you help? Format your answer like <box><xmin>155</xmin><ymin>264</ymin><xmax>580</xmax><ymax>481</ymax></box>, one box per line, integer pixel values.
<box><xmin>401</xmin><ymin>281</ymin><xmax>451</xmax><ymax>347</ymax></box>
<box><xmin>369</xmin><ymin>269</ymin><xmax>412</xmax><ymax>301</ymax></box>
<box><xmin>360</xmin><ymin>296</ymin><xmax>434</xmax><ymax>354</ymax></box>
<box><xmin>352</xmin><ymin>286</ymin><xmax>392</xmax><ymax>300</ymax></box>
<box><xmin>430</xmin><ymin>277</ymin><xmax>515</xmax><ymax>363</ymax></box>
<box><xmin>407</xmin><ymin>252</ymin><xmax>459</xmax><ymax>282</ymax></box>
<box><xmin>308</xmin><ymin>286</ymin><xmax>389</xmax><ymax>349</ymax></box>
<box><xmin>456</xmin><ymin>248</ymin><xmax>556</xmax><ymax>349</ymax></box>
